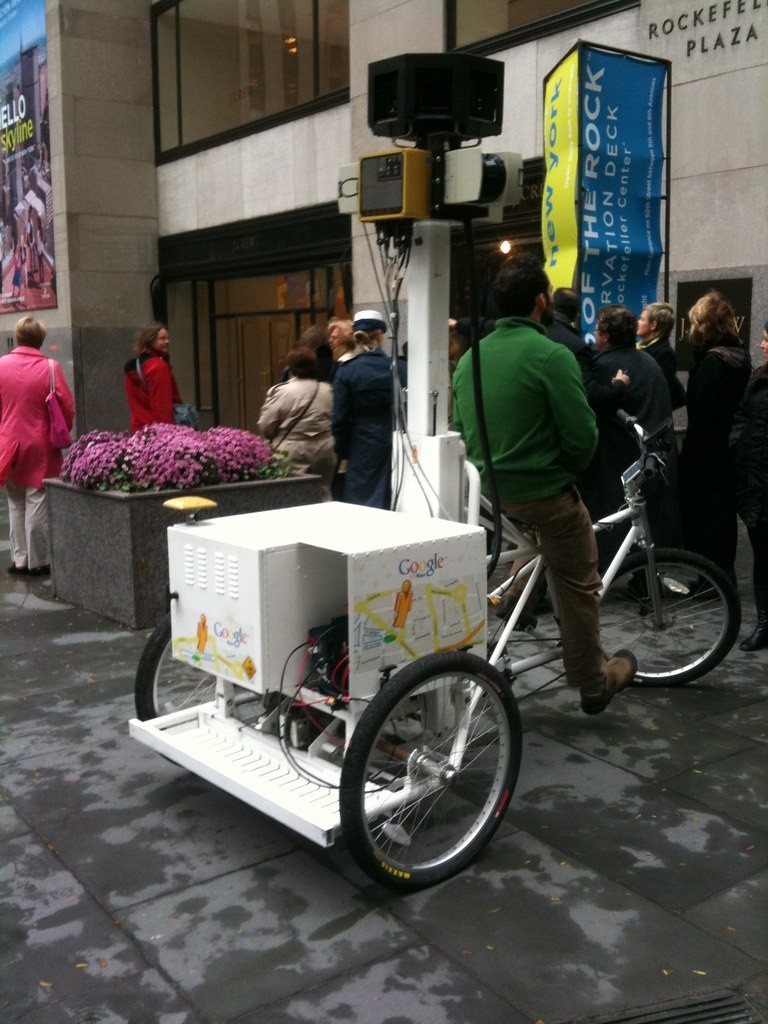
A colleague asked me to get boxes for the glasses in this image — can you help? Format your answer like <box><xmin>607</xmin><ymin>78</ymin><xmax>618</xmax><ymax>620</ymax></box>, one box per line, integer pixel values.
<box><xmin>590</xmin><ymin>326</ymin><xmax>598</xmax><ymax>333</ymax></box>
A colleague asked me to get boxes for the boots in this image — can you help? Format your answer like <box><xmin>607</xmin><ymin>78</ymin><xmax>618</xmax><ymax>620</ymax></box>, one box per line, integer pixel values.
<box><xmin>739</xmin><ymin>585</ymin><xmax>768</xmax><ymax>651</ymax></box>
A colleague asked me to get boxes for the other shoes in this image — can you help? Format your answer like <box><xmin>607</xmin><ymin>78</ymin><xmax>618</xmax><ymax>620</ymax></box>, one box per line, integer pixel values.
<box><xmin>694</xmin><ymin>590</ymin><xmax>721</xmax><ymax>602</ymax></box>
<box><xmin>625</xmin><ymin>575</ymin><xmax>662</xmax><ymax>601</ymax></box>
<box><xmin>29</xmin><ymin>564</ymin><xmax>50</xmax><ymax>577</ymax></box>
<box><xmin>580</xmin><ymin>650</ymin><xmax>638</xmax><ymax>716</ymax></box>
<box><xmin>495</xmin><ymin>589</ymin><xmax>538</xmax><ymax>631</ymax></box>
<box><xmin>7</xmin><ymin>563</ymin><xmax>29</xmax><ymax>575</ymax></box>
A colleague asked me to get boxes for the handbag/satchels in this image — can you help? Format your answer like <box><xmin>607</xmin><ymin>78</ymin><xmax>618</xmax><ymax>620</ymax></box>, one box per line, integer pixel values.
<box><xmin>173</xmin><ymin>403</ymin><xmax>199</xmax><ymax>428</ymax></box>
<box><xmin>45</xmin><ymin>358</ymin><xmax>73</xmax><ymax>448</ymax></box>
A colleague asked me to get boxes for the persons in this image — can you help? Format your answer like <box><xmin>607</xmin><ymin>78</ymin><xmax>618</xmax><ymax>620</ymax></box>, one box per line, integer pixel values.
<box><xmin>9</xmin><ymin>206</ymin><xmax>46</xmax><ymax>298</ymax></box>
<box><xmin>1</xmin><ymin>316</ymin><xmax>76</xmax><ymax>577</ymax></box>
<box><xmin>257</xmin><ymin>288</ymin><xmax>768</xmax><ymax>652</ymax></box>
<box><xmin>120</xmin><ymin>322</ymin><xmax>183</xmax><ymax>434</ymax></box>
<box><xmin>451</xmin><ymin>255</ymin><xmax>638</xmax><ymax>716</ymax></box>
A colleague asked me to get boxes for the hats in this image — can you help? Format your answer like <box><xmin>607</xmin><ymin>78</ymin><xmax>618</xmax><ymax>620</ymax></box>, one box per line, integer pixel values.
<box><xmin>352</xmin><ymin>309</ymin><xmax>387</xmax><ymax>333</ymax></box>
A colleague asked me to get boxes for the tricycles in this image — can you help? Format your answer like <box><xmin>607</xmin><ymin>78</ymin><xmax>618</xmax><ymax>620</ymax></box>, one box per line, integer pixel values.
<box><xmin>127</xmin><ymin>406</ymin><xmax>742</xmax><ymax>897</ymax></box>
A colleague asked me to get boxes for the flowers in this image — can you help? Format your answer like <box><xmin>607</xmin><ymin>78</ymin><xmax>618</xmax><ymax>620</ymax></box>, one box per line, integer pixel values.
<box><xmin>61</xmin><ymin>423</ymin><xmax>292</xmax><ymax>491</ymax></box>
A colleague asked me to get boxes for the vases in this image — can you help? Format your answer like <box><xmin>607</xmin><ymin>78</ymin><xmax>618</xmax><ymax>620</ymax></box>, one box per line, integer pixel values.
<box><xmin>42</xmin><ymin>476</ymin><xmax>329</xmax><ymax>629</ymax></box>
